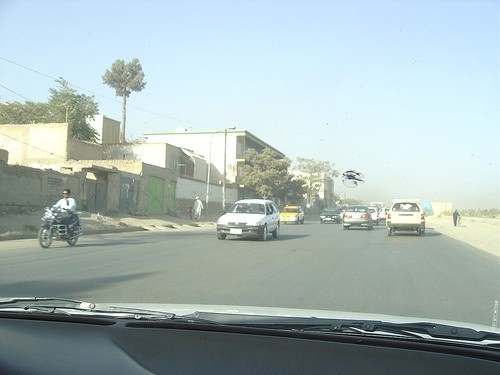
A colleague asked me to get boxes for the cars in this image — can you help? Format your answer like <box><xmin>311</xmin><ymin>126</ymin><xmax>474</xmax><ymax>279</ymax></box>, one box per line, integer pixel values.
<box><xmin>343</xmin><ymin>205</ymin><xmax>373</xmax><ymax>230</ymax></box>
<box><xmin>217</xmin><ymin>199</ymin><xmax>280</xmax><ymax>240</ymax></box>
<box><xmin>320</xmin><ymin>208</ymin><xmax>340</xmax><ymax>224</ymax></box>
<box><xmin>280</xmin><ymin>205</ymin><xmax>304</xmax><ymax>225</ymax></box>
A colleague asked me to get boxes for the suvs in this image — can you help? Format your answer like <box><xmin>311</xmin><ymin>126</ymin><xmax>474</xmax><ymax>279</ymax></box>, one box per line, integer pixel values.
<box><xmin>386</xmin><ymin>201</ymin><xmax>425</xmax><ymax>236</ymax></box>
<box><xmin>369</xmin><ymin>202</ymin><xmax>389</xmax><ymax>225</ymax></box>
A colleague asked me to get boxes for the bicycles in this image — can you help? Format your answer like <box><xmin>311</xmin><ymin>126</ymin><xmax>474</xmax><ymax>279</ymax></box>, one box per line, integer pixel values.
<box><xmin>457</xmin><ymin>216</ymin><xmax>462</xmax><ymax>227</ymax></box>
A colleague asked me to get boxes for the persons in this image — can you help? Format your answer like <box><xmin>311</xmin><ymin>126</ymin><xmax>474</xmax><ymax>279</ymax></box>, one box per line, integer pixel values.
<box><xmin>188</xmin><ymin>207</ymin><xmax>193</xmax><ymax>220</ymax></box>
<box><xmin>51</xmin><ymin>189</ymin><xmax>77</xmax><ymax>239</ymax></box>
<box><xmin>453</xmin><ymin>210</ymin><xmax>459</xmax><ymax>226</ymax></box>
<box><xmin>193</xmin><ymin>197</ymin><xmax>203</xmax><ymax>220</ymax></box>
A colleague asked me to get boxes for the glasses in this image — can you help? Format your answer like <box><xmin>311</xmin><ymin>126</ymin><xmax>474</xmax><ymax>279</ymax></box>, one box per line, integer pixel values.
<box><xmin>63</xmin><ymin>193</ymin><xmax>69</xmax><ymax>195</ymax></box>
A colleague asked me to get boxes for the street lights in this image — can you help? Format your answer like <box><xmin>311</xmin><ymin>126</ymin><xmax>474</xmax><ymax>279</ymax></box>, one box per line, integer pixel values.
<box><xmin>222</xmin><ymin>126</ymin><xmax>236</xmax><ymax>213</ymax></box>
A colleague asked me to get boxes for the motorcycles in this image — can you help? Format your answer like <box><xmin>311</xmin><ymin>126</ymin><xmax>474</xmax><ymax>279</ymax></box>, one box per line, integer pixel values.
<box><xmin>38</xmin><ymin>208</ymin><xmax>80</xmax><ymax>248</ymax></box>
